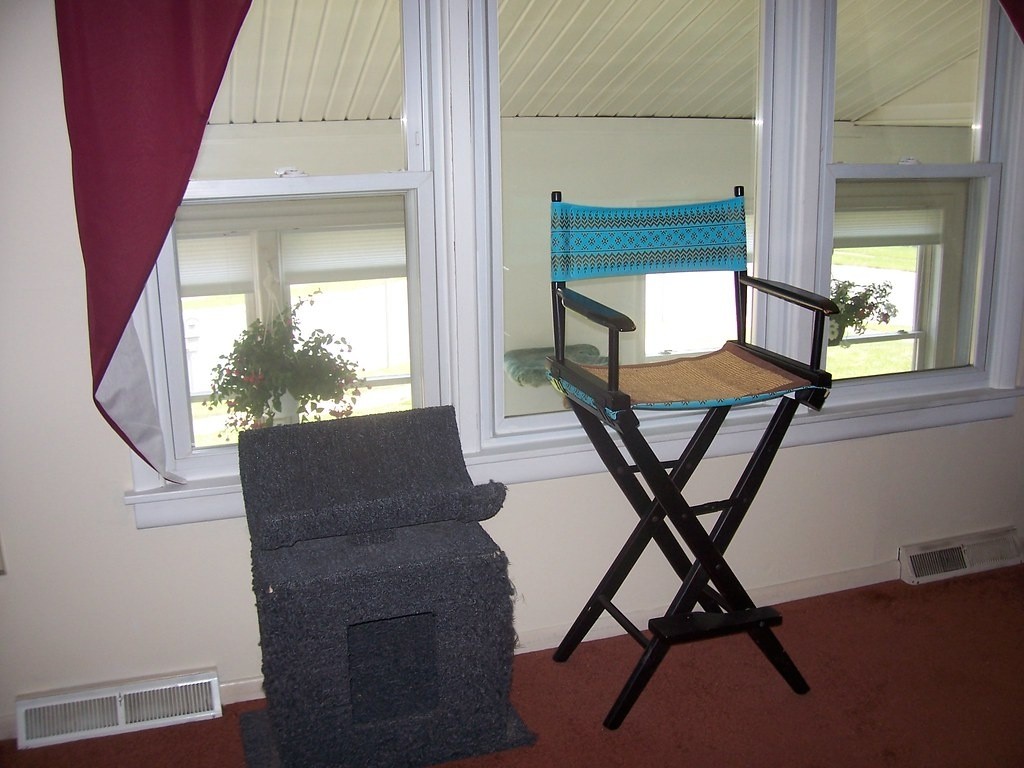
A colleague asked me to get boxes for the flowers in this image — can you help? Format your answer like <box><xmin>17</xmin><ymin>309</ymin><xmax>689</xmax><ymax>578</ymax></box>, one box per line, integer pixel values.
<box><xmin>827</xmin><ymin>279</ymin><xmax>899</xmax><ymax>348</ymax></box>
<box><xmin>203</xmin><ymin>296</ymin><xmax>372</xmax><ymax>441</ymax></box>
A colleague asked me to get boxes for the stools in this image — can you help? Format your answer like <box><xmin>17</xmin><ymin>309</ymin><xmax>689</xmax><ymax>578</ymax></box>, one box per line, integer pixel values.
<box><xmin>545</xmin><ymin>185</ymin><xmax>834</xmax><ymax>732</ymax></box>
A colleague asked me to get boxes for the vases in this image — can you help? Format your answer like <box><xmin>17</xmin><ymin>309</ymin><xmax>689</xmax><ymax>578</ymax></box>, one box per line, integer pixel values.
<box><xmin>262</xmin><ymin>386</ymin><xmax>302</xmax><ymax>421</ymax></box>
<box><xmin>826</xmin><ymin>316</ymin><xmax>851</xmax><ymax>345</ymax></box>
